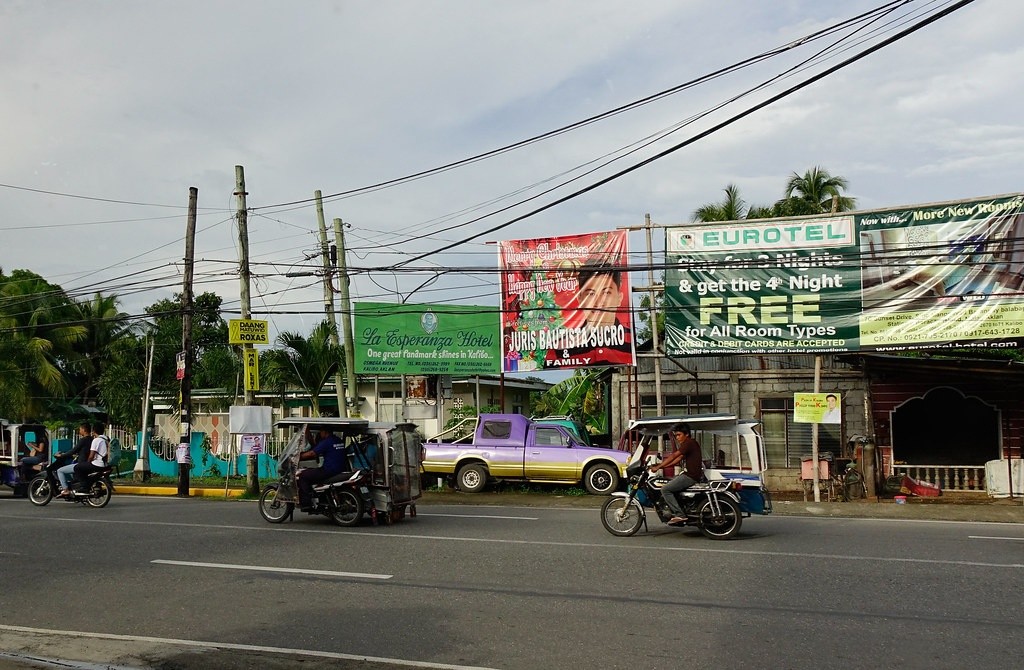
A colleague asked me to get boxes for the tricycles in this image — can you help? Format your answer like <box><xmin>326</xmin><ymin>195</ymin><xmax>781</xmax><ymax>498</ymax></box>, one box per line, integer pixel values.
<box><xmin>0</xmin><ymin>420</ymin><xmax>52</xmax><ymax>496</ymax></box>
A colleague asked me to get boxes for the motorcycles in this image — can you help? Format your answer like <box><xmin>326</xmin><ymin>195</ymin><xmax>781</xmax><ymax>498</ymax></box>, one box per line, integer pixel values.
<box><xmin>27</xmin><ymin>452</ymin><xmax>116</xmax><ymax>508</ymax></box>
<box><xmin>257</xmin><ymin>415</ymin><xmax>422</xmax><ymax>527</ymax></box>
<box><xmin>597</xmin><ymin>413</ymin><xmax>773</xmax><ymax>541</ymax></box>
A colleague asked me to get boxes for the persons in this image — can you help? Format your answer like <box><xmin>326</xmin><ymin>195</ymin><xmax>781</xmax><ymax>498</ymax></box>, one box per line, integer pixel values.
<box><xmin>347</xmin><ymin>433</ymin><xmax>381</xmax><ymax>474</ymax></box>
<box><xmin>553</xmin><ymin>258</ymin><xmax>633</xmax><ymax>368</ymax></box>
<box><xmin>286</xmin><ymin>424</ymin><xmax>349</xmax><ymax>510</ymax></box>
<box><xmin>54</xmin><ymin>422</ymin><xmax>95</xmax><ymax>499</ymax></box>
<box><xmin>650</xmin><ymin>423</ymin><xmax>704</xmax><ymax>526</ymax></box>
<box><xmin>14</xmin><ymin>426</ymin><xmax>50</xmax><ymax>482</ymax></box>
<box><xmin>6</xmin><ymin>432</ymin><xmax>31</xmax><ymax>461</ymax></box>
<box><xmin>73</xmin><ymin>422</ymin><xmax>111</xmax><ymax>493</ymax></box>
<box><xmin>821</xmin><ymin>394</ymin><xmax>841</xmax><ymax>424</ymax></box>
<box><xmin>182</xmin><ymin>447</ymin><xmax>190</xmax><ymax>463</ymax></box>
<box><xmin>250</xmin><ymin>436</ymin><xmax>263</xmax><ymax>453</ymax></box>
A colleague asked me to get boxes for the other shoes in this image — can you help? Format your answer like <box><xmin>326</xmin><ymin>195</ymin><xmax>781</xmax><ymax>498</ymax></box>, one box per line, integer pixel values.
<box><xmin>295</xmin><ymin>500</ymin><xmax>314</xmax><ymax>509</ymax></box>
<box><xmin>55</xmin><ymin>492</ymin><xmax>70</xmax><ymax>499</ymax></box>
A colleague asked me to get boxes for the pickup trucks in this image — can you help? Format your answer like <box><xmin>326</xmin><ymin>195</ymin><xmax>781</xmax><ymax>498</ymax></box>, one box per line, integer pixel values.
<box><xmin>416</xmin><ymin>413</ymin><xmax>631</xmax><ymax>496</ymax></box>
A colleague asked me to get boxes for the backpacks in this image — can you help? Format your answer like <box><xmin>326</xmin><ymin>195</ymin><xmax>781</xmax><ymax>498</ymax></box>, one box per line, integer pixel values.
<box><xmin>94</xmin><ymin>435</ymin><xmax>121</xmax><ymax>467</ymax></box>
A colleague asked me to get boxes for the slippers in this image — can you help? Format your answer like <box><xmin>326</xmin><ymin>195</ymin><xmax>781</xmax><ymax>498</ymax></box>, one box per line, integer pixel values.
<box><xmin>668</xmin><ymin>516</ymin><xmax>688</xmax><ymax>524</ymax></box>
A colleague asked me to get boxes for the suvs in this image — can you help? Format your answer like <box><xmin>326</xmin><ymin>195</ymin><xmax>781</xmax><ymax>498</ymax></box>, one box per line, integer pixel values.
<box><xmin>531</xmin><ymin>415</ymin><xmax>592</xmax><ymax>449</ymax></box>
